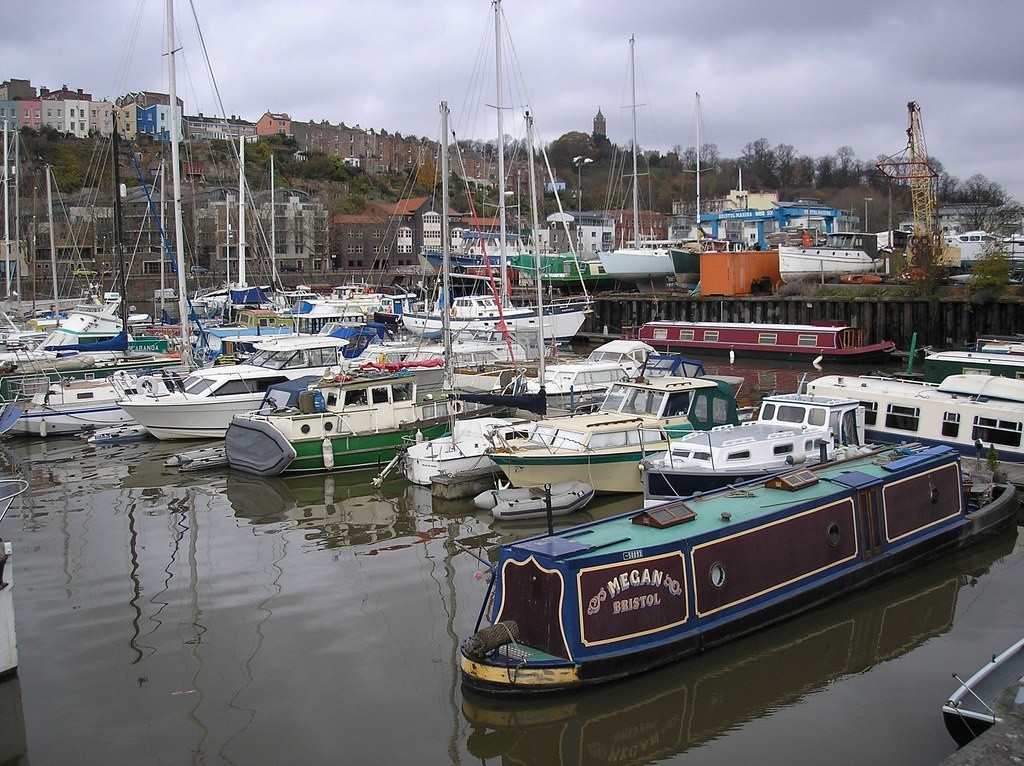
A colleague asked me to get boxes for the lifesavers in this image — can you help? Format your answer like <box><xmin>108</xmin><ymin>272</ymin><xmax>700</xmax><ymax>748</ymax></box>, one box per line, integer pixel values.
<box><xmin>450</xmin><ymin>307</ymin><xmax>457</xmax><ymax>316</ymax></box>
<box><xmin>348</xmin><ymin>292</ymin><xmax>353</xmax><ymax>299</ymax></box>
<box><xmin>135</xmin><ymin>375</ymin><xmax>158</xmax><ymax>394</ymax></box>
<box><xmin>379</xmin><ymin>353</ymin><xmax>386</xmax><ymax>364</ymax></box>
<box><xmin>334</xmin><ymin>373</ymin><xmax>353</xmax><ymax>381</ymax></box>
<box><xmin>113</xmin><ymin>370</ymin><xmax>132</xmax><ymax>389</ymax></box>
<box><xmin>911</xmin><ymin>236</ymin><xmax>930</xmax><ymax>265</ymax></box>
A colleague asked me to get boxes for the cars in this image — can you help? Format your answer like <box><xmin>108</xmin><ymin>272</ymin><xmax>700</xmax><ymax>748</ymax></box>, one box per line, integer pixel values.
<box><xmin>280</xmin><ymin>265</ymin><xmax>300</xmax><ymax>272</ymax></box>
<box><xmin>103</xmin><ymin>267</ymin><xmax>121</xmax><ymax>276</ymax></box>
<box><xmin>72</xmin><ymin>267</ymin><xmax>98</xmax><ymax>277</ymax></box>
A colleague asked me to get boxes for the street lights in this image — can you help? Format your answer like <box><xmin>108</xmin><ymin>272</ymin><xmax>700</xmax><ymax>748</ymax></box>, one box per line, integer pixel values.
<box><xmin>864</xmin><ymin>197</ymin><xmax>872</xmax><ymax>233</ymax></box>
<box><xmin>573</xmin><ymin>156</ymin><xmax>595</xmax><ymax>261</ymax></box>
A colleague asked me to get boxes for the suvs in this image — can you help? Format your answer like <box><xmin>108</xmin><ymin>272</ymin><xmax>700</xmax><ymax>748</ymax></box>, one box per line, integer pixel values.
<box><xmin>190</xmin><ymin>265</ymin><xmax>209</xmax><ymax>274</ymax></box>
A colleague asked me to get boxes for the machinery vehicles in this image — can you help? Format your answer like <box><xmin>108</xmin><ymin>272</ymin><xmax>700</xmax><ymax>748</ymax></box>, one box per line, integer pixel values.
<box><xmin>874</xmin><ymin>100</ymin><xmax>961</xmax><ymax>285</ymax></box>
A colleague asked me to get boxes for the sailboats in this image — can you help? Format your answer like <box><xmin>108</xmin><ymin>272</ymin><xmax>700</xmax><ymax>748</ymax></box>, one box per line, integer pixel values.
<box><xmin>1</xmin><ymin>0</ymin><xmax>1023</xmax><ymax>493</ymax></box>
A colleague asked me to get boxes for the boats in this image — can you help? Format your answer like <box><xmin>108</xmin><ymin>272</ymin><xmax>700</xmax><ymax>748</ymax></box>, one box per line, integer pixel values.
<box><xmin>162</xmin><ymin>447</ymin><xmax>230</xmax><ymax>472</ymax></box>
<box><xmin>636</xmin><ymin>371</ymin><xmax>869</xmax><ymax>509</ymax></box>
<box><xmin>940</xmin><ymin>638</ymin><xmax>1024</xmax><ymax>747</ymax></box>
<box><xmin>458</xmin><ymin>437</ymin><xmax>1024</xmax><ymax>700</ymax></box>
<box><xmin>452</xmin><ymin>524</ymin><xmax>1020</xmax><ymax>766</ymax></box>
<box><xmin>79</xmin><ymin>421</ymin><xmax>148</xmax><ymax>447</ymax></box>
<box><xmin>469</xmin><ymin>478</ymin><xmax>595</xmax><ymax>521</ymax></box>
<box><xmin>0</xmin><ymin>477</ymin><xmax>31</xmax><ymax>678</ymax></box>
